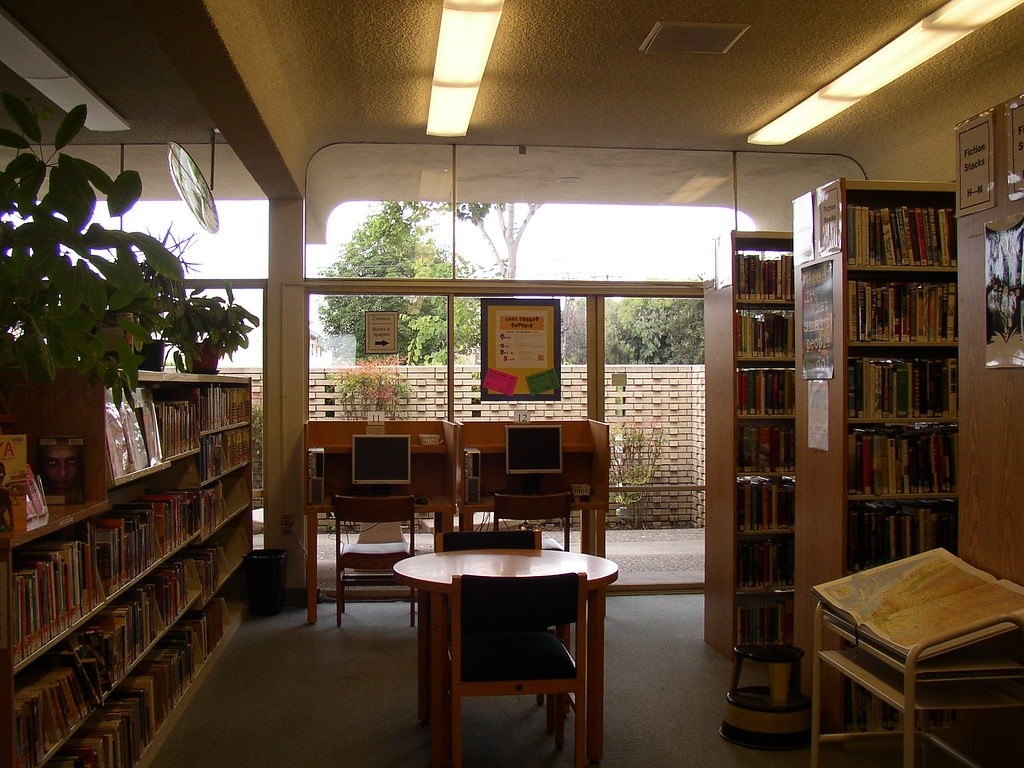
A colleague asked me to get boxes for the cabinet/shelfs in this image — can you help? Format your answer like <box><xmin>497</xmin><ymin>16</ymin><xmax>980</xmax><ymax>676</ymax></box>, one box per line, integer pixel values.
<box><xmin>810</xmin><ymin>601</ymin><xmax>1024</xmax><ymax>768</ymax></box>
<box><xmin>954</xmin><ymin>90</ymin><xmax>1024</xmax><ymax>768</ymax></box>
<box><xmin>0</xmin><ymin>367</ymin><xmax>255</xmax><ymax>768</ymax></box>
<box><xmin>703</xmin><ymin>230</ymin><xmax>796</xmax><ymax>660</ymax></box>
<box><xmin>793</xmin><ymin>176</ymin><xmax>957</xmax><ymax>750</ymax></box>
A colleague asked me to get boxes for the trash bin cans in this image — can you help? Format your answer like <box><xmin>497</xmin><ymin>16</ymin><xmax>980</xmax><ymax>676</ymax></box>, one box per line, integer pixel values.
<box><xmin>245</xmin><ymin>549</ymin><xmax>288</xmax><ymax>617</ymax></box>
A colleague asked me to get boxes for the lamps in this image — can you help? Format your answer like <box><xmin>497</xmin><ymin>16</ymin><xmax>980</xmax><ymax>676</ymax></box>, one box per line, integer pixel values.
<box><xmin>0</xmin><ymin>8</ymin><xmax>80</xmax><ymax>79</ymax></box>
<box><xmin>26</xmin><ymin>71</ymin><xmax>132</xmax><ymax>133</ymax></box>
<box><xmin>743</xmin><ymin>0</ymin><xmax>1024</xmax><ymax>146</ymax></box>
<box><xmin>426</xmin><ymin>0</ymin><xmax>506</xmax><ymax>137</ymax></box>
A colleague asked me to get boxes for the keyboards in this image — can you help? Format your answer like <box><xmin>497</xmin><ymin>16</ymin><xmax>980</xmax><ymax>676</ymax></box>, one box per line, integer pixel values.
<box><xmin>568</xmin><ymin>495</ymin><xmax>575</xmax><ymax>504</ymax></box>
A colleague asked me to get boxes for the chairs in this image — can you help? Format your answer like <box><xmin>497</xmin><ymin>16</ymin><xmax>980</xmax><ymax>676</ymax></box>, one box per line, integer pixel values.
<box><xmin>332</xmin><ymin>493</ymin><xmax>417</xmax><ymax>629</ymax></box>
<box><xmin>449</xmin><ymin>573</ymin><xmax>589</xmax><ymax>768</ymax></box>
<box><xmin>492</xmin><ymin>491</ymin><xmax>573</xmax><ymax>552</ymax></box>
<box><xmin>434</xmin><ymin>530</ymin><xmax>555</xmax><ymax>735</ymax></box>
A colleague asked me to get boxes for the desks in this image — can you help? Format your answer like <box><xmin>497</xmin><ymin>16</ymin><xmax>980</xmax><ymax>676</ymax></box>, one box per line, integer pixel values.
<box><xmin>391</xmin><ymin>548</ymin><xmax>620</xmax><ymax>763</ymax></box>
<box><xmin>455</xmin><ymin>419</ymin><xmax>611</xmax><ymax>619</ymax></box>
<box><xmin>302</xmin><ymin>420</ymin><xmax>459</xmax><ymax>624</ymax></box>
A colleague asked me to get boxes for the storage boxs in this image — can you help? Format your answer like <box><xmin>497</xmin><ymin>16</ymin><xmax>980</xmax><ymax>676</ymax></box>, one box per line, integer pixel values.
<box><xmin>308</xmin><ymin>447</ymin><xmax>324</xmax><ymax>479</ymax></box>
<box><xmin>419</xmin><ymin>434</ymin><xmax>440</xmax><ymax>446</ymax></box>
<box><xmin>464</xmin><ymin>447</ymin><xmax>481</xmax><ymax>479</ymax></box>
<box><xmin>465</xmin><ymin>479</ymin><xmax>481</xmax><ymax>505</ymax></box>
<box><xmin>308</xmin><ymin>478</ymin><xmax>325</xmax><ymax>507</ymax></box>
<box><xmin>570</xmin><ymin>483</ymin><xmax>591</xmax><ymax>496</ymax></box>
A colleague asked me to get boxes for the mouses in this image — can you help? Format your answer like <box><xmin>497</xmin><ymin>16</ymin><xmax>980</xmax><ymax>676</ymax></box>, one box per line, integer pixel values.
<box><xmin>416</xmin><ymin>498</ymin><xmax>428</xmax><ymax>505</ymax></box>
<box><xmin>579</xmin><ymin>494</ymin><xmax>591</xmax><ymax>503</ymax></box>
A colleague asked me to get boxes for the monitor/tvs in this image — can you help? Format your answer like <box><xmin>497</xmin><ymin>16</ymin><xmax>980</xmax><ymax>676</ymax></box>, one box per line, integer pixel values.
<box><xmin>505</xmin><ymin>424</ymin><xmax>562</xmax><ymax>490</ymax></box>
<box><xmin>352</xmin><ymin>434</ymin><xmax>411</xmax><ymax>497</ymax></box>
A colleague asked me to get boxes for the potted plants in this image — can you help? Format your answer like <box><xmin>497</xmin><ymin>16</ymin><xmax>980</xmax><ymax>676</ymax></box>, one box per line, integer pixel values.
<box><xmin>173</xmin><ymin>324</ymin><xmax>174</xmax><ymax>327</ymax></box>
<box><xmin>0</xmin><ymin>95</ymin><xmax>260</xmax><ymax>410</ymax></box>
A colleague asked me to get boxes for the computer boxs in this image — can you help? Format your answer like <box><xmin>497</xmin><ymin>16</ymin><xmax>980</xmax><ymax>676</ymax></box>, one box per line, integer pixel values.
<box><xmin>464</xmin><ymin>448</ymin><xmax>481</xmax><ymax>505</ymax></box>
<box><xmin>307</xmin><ymin>448</ymin><xmax>325</xmax><ymax>505</ymax></box>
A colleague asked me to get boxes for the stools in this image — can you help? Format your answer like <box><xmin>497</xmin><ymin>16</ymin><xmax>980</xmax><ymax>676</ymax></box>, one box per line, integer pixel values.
<box><xmin>718</xmin><ymin>644</ymin><xmax>812</xmax><ymax>751</ymax></box>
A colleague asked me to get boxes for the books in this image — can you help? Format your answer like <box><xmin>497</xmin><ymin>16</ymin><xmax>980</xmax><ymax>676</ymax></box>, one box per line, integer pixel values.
<box><xmin>0</xmin><ymin>388</ymin><xmax>249</xmax><ymax>768</ymax></box>
<box><xmin>734</xmin><ymin>255</ymin><xmax>794</xmax><ymax>645</ymax></box>
<box><xmin>811</xmin><ymin>547</ymin><xmax>1024</xmax><ymax>662</ymax></box>
<box><xmin>844</xmin><ymin>205</ymin><xmax>958</xmax><ymax>571</ymax></box>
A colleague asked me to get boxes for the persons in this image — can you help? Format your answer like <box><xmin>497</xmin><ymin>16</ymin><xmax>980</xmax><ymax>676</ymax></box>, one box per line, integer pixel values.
<box><xmin>44</xmin><ymin>440</ymin><xmax>78</xmax><ymax>494</ymax></box>
